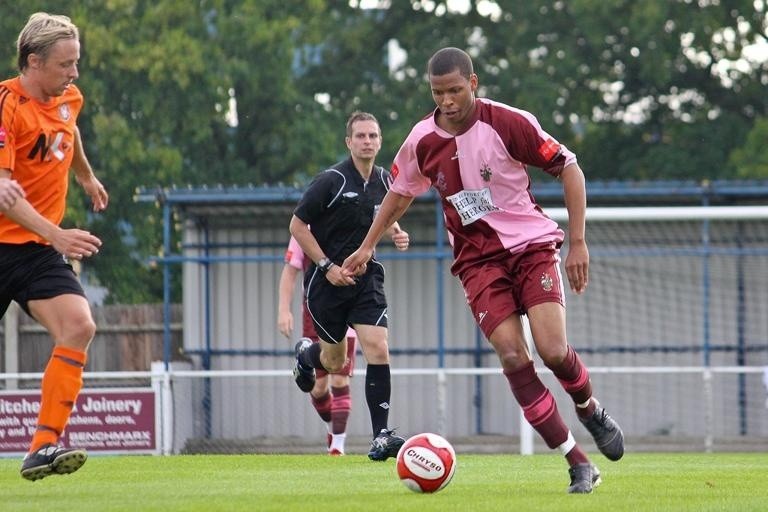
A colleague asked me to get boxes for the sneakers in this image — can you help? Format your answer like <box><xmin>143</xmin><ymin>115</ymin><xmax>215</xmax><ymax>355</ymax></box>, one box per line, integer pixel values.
<box><xmin>293</xmin><ymin>337</ymin><xmax>317</xmax><ymax>392</ymax></box>
<box><xmin>20</xmin><ymin>442</ymin><xmax>87</xmax><ymax>481</ymax></box>
<box><xmin>568</xmin><ymin>464</ymin><xmax>603</xmax><ymax>494</ymax></box>
<box><xmin>578</xmin><ymin>397</ymin><xmax>624</xmax><ymax>461</ymax></box>
<box><xmin>368</xmin><ymin>428</ymin><xmax>406</xmax><ymax>460</ymax></box>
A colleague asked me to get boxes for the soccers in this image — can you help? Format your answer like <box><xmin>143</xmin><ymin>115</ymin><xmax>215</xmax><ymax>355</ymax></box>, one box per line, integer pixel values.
<box><xmin>396</xmin><ymin>434</ymin><xmax>457</xmax><ymax>494</ymax></box>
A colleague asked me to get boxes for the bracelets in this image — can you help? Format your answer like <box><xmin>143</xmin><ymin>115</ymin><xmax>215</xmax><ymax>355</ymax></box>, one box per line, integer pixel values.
<box><xmin>320</xmin><ymin>261</ymin><xmax>334</xmax><ymax>277</ymax></box>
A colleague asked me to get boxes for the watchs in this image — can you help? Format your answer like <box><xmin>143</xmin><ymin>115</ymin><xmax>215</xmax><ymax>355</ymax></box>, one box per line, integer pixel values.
<box><xmin>316</xmin><ymin>256</ymin><xmax>331</xmax><ymax>274</ymax></box>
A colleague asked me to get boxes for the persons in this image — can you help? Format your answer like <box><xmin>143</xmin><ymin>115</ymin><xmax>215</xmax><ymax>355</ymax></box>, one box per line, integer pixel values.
<box><xmin>277</xmin><ymin>202</ymin><xmax>409</xmax><ymax>456</ymax></box>
<box><xmin>0</xmin><ymin>11</ymin><xmax>109</xmax><ymax>481</ymax></box>
<box><xmin>341</xmin><ymin>48</ymin><xmax>624</xmax><ymax>492</ymax></box>
<box><xmin>0</xmin><ymin>176</ymin><xmax>27</xmax><ymax>213</ymax></box>
<box><xmin>288</xmin><ymin>111</ymin><xmax>409</xmax><ymax>462</ymax></box>
